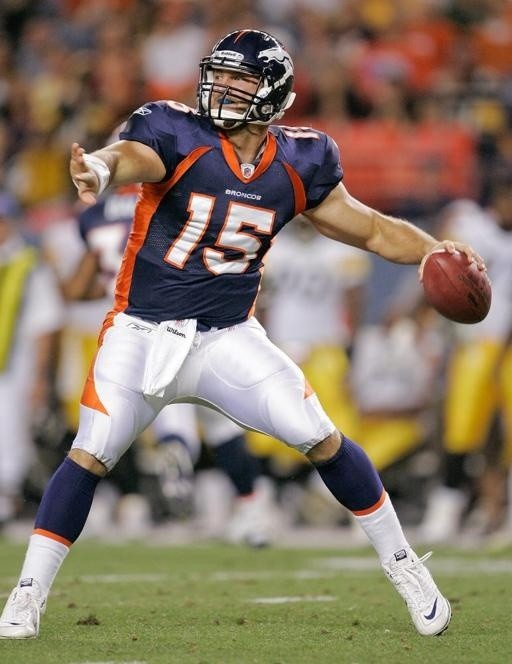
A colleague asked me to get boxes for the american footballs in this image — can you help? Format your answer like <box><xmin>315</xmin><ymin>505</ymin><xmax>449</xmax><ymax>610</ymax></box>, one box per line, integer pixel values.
<box><xmin>423</xmin><ymin>249</ymin><xmax>491</xmax><ymax>324</ymax></box>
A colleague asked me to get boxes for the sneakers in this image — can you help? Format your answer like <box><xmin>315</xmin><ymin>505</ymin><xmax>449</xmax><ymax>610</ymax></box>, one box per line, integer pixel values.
<box><xmin>379</xmin><ymin>542</ymin><xmax>452</xmax><ymax>637</ymax></box>
<box><xmin>1</xmin><ymin>576</ymin><xmax>48</xmax><ymax>640</ymax></box>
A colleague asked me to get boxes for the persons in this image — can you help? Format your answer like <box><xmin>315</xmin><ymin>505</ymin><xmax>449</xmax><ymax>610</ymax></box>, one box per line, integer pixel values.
<box><xmin>1</xmin><ymin>29</ymin><xmax>486</xmax><ymax>638</ymax></box>
<box><xmin>1</xmin><ymin>1</ymin><xmax>510</xmax><ymax>553</ymax></box>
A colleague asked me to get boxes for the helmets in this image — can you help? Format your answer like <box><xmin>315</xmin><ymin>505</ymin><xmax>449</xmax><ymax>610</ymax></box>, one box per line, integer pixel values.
<box><xmin>194</xmin><ymin>28</ymin><xmax>296</xmax><ymax>130</ymax></box>
